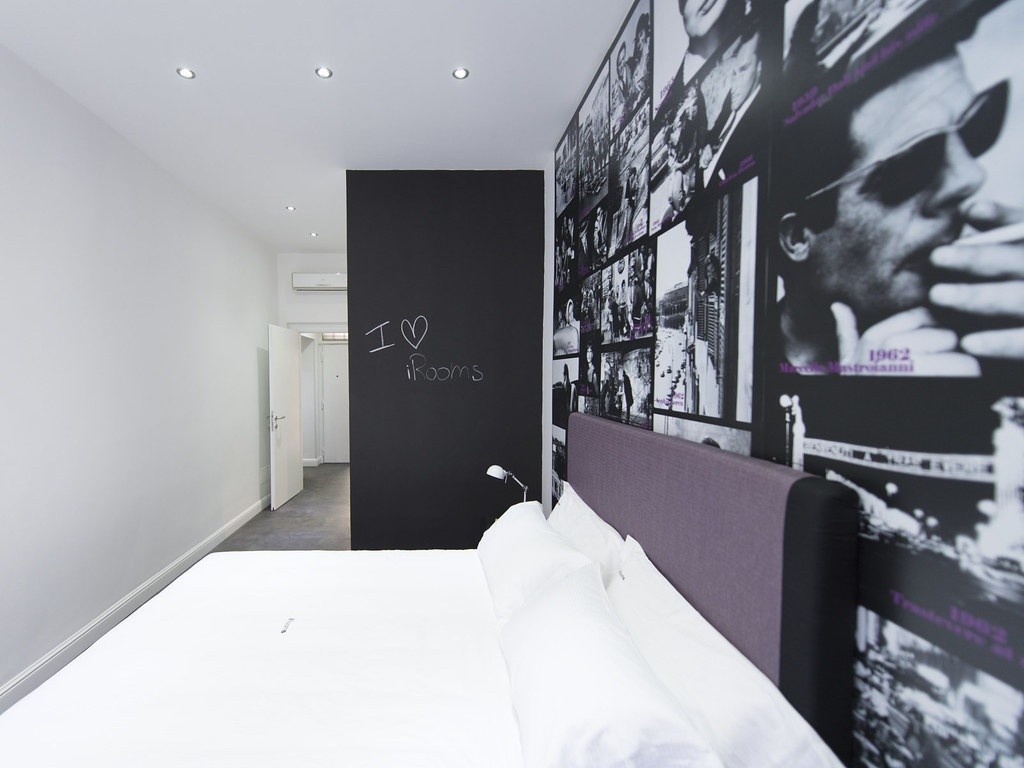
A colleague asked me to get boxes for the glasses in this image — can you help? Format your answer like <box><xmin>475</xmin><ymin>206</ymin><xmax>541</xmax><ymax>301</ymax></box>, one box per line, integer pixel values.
<box><xmin>796</xmin><ymin>82</ymin><xmax>1009</xmax><ymax>210</ymax></box>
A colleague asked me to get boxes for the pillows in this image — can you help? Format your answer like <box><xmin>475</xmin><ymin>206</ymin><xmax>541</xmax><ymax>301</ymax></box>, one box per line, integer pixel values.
<box><xmin>476</xmin><ymin>480</ymin><xmax>847</xmax><ymax>768</ymax></box>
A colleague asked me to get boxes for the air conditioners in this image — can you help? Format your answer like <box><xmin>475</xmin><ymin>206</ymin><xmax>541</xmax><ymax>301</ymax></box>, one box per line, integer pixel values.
<box><xmin>292</xmin><ymin>272</ymin><xmax>348</xmax><ymax>294</ymax></box>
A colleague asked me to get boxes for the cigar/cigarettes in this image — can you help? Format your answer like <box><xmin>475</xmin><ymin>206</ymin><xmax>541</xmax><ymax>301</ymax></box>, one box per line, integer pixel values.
<box><xmin>953</xmin><ymin>220</ymin><xmax>1024</xmax><ymax>245</ymax></box>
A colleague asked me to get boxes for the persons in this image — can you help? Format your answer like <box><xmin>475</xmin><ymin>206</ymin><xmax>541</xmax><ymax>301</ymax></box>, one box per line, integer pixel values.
<box><xmin>611</xmin><ymin>13</ymin><xmax>649</xmax><ymax>98</ymax></box>
<box><xmin>653</xmin><ymin>0</ymin><xmax>748</xmax><ymax>124</ymax></box>
<box><xmin>698</xmin><ymin>33</ymin><xmax>763</xmax><ymax>183</ymax></box>
<box><xmin>766</xmin><ymin>33</ymin><xmax>1024</xmax><ymax>376</ymax></box>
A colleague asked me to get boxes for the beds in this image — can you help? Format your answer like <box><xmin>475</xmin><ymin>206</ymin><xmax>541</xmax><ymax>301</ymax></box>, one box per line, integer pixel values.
<box><xmin>0</xmin><ymin>413</ymin><xmax>863</xmax><ymax>768</ymax></box>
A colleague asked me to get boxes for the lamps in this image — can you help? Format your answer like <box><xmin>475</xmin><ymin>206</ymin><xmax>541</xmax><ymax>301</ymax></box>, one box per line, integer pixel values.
<box><xmin>487</xmin><ymin>465</ymin><xmax>528</xmax><ymax>503</ymax></box>
